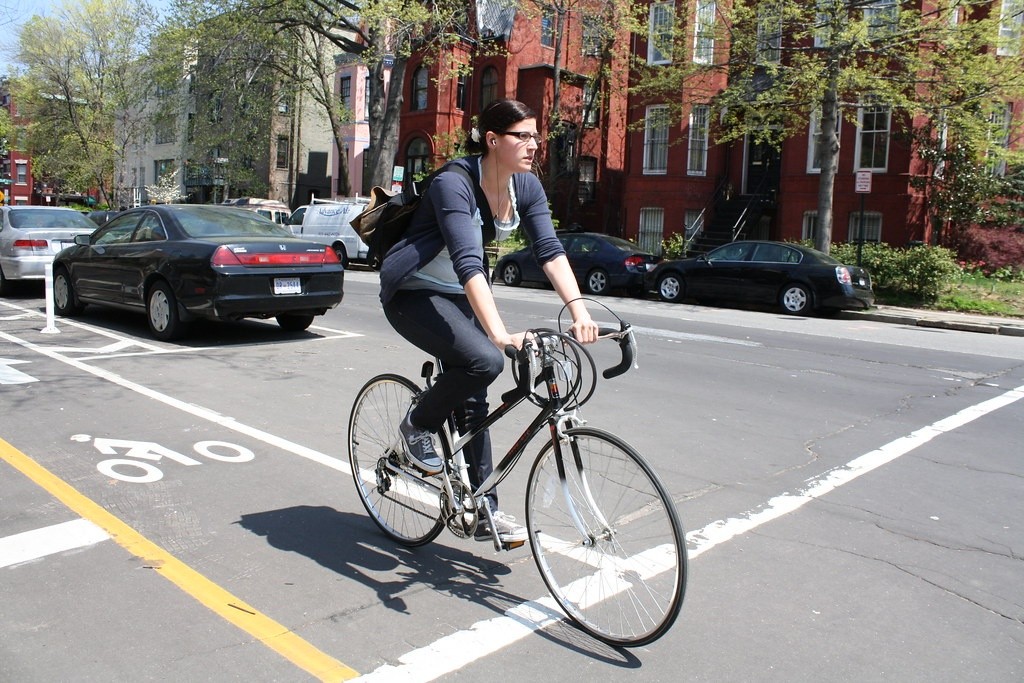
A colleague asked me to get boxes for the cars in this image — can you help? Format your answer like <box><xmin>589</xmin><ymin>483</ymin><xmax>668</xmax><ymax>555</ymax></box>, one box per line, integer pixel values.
<box><xmin>644</xmin><ymin>241</ymin><xmax>876</xmax><ymax>316</ymax></box>
<box><xmin>494</xmin><ymin>233</ymin><xmax>664</xmax><ymax>296</ymax></box>
<box><xmin>52</xmin><ymin>203</ymin><xmax>345</xmax><ymax>340</ymax></box>
<box><xmin>85</xmin><ymin>211</ymin><xmax>119</xmax><ymax>226</ymax></box>
<box><xmin>0</xmin><ymin>205</ymin><xmax>114</xmax><ymax>292</ymax></box>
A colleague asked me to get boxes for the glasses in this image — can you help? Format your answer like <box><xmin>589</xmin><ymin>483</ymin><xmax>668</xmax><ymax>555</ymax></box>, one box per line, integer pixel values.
<box><xmin>493</xmin><ymin>130</ymin><xmax>542</xmax><ymax>143</ymax></box>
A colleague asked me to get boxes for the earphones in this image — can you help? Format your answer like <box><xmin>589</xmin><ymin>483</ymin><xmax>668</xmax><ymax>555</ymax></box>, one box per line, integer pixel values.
<box><xmin>491</xmin><ymin>138</ymin><xmax>498</xmax><ymax>147</ymax></box>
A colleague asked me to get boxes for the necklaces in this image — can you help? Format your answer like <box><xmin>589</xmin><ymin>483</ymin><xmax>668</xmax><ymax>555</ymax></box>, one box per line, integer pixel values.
<box><xmin>482</xmin><ymin>178</ymin><xmax>508</xmax><ymax>219</ymax></box>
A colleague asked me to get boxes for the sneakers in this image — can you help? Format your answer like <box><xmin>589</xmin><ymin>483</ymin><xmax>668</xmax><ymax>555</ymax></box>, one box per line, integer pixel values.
<box><xmin>474</xmin><ymin>516</ymin><xmax>532</xmax><ymax>542</ymax></box>
<box><xmin>397</xmin><ymin>412</ymin><xmax>443</xmax><ymax>472</ymax></box>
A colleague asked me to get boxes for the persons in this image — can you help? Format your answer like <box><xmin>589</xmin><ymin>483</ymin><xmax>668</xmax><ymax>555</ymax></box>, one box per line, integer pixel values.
<box><xmin>379</xmin><ymin>99</ymin><xmax>599</xmax><ymax>544</ymax></box>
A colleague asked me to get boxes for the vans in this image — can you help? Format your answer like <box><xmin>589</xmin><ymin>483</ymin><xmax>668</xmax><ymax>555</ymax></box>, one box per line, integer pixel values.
<box><xmin>221</xmin><ymin>199</ymin><xmax>290</xmax><ymax>230</ymax></box>
<box><xmin>278</xmin><ymin>203</ymin><xmax>385</xmax><ymax>270</ymax></box>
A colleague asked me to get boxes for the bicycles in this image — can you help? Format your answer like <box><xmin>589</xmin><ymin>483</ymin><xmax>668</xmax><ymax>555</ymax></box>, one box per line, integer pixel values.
<box><xmin>347</xmin><ymin>297</ymin><xmax>689</xmax><ymax>648</ymax></box>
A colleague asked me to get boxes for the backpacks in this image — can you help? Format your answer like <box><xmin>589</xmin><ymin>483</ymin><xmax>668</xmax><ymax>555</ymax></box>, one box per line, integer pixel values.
<box><xmin>350</xmin><ymin>158</ymin><xmax>494</xmax><ymax>257</ymax></box>
